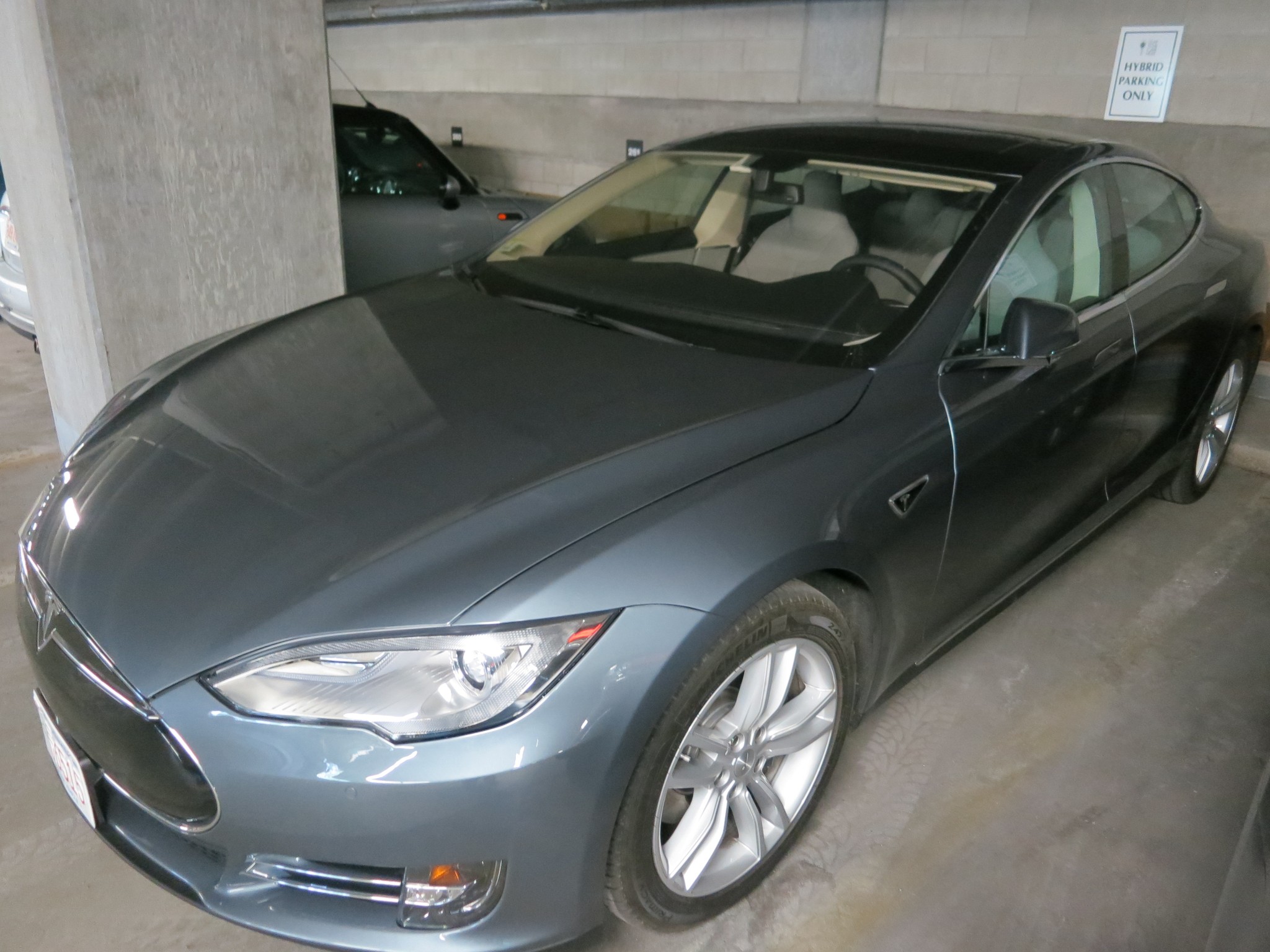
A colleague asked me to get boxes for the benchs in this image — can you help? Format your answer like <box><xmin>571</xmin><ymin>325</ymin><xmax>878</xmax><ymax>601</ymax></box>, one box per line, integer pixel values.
<box><xmin>860</xmin><ymin>191</ymin><xmax>1161</xmax><ymax>315</ymax></box>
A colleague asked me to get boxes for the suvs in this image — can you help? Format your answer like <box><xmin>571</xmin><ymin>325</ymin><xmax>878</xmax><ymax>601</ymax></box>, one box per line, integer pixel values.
<box><xmin>1</xmin><ymin>103</ymin><xmax>584</xmax><ymax>358</ymax></box>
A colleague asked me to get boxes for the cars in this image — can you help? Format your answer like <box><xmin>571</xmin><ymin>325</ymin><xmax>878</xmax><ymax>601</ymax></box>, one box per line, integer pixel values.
<box><xmin>13</xmin><ymin>115</ymin><xmax>1269</xmax><ymax>952</ymax></box>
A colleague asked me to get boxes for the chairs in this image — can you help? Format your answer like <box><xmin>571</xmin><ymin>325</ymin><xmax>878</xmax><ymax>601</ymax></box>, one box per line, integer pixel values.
<box><xmin>917</xmin><ymin>194</ymin><xmax>1061</xmax><ymax>336</ymax></box>
<box><xmin>721</xmin><ymin>167</ymin><xmax>862</xmax><ymax>284</ymax></box>
